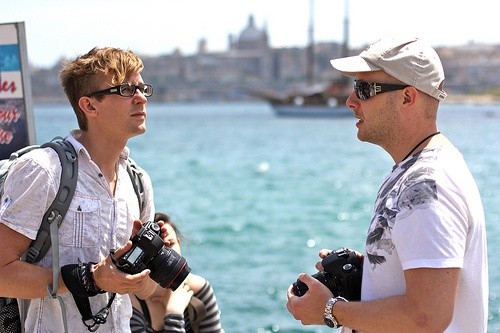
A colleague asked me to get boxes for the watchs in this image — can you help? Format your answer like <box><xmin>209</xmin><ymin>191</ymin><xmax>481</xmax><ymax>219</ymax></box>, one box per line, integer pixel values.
<box><xmin>323</xmin><ymin>296</ymin><xmax>349</xmax><ymax>329</ymax></box>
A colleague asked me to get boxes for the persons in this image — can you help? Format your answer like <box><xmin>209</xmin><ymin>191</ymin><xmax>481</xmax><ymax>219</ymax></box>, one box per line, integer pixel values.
<box><xmin>1</xmin><ymin>47</ymin><xmax>170</xmax><ymax>333</ymax></box>
<box><xmin>288</xmin><ymin>37</ymin><xmax>488</xmax><ymax>332</ymax></box>
<box><xmin>130</xmin><ymin>213</ymin><xmax>222</xmax><ymax>333</ymax></box>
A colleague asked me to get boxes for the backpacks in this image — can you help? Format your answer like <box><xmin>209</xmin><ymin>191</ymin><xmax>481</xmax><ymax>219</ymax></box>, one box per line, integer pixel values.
<box><xmin>0</xmin><ymin>136</ymin><xmax>145</xmax><ymax>333</ymax></box>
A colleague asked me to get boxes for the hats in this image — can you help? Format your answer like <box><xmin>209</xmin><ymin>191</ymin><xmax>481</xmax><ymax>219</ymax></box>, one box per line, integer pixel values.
<box><xmin>330</xmin><ymin>35</ymin><xmax>447</xmax><ymax>101</ymax></box>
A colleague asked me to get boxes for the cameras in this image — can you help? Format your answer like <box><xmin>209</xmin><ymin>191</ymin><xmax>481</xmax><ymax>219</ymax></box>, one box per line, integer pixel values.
<box><xmin>117</xmin><ymin>221</ymin><xmax>192</xmax><ymax>292</ymax></box>
<box><xmin>292</xmin><ymin>247</ymin><xmax>363</xmax><ymax>301</ymax></box>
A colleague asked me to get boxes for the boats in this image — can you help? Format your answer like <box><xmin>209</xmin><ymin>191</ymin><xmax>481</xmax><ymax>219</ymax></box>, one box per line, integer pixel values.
<box><xmin>232</xmin><ymin>72</ymin><xmax>359</xmax><ymax>117</ymax></box>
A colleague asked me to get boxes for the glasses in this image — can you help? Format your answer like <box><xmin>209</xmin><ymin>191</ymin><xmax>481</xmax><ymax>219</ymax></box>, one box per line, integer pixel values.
<box><xmin>353</xmin><ymin>80</ymin><xmax>409</xmax><ymax>101</ymax></box>
<box><xmin>73</xmin><ymin>83</ymin><xmax>153</xmax><ymax>107</ymax></box>
